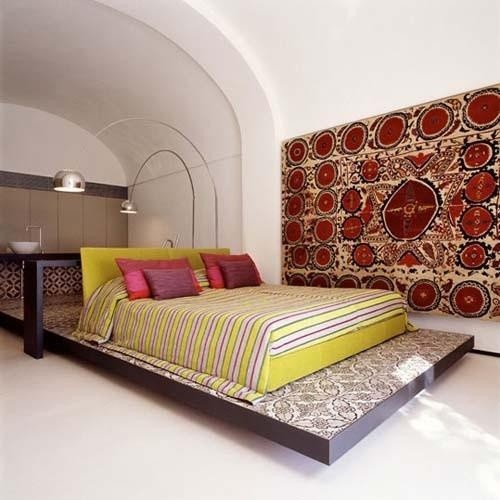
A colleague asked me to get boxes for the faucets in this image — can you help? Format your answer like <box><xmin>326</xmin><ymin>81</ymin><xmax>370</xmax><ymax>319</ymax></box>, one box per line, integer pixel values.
<box><xmin>24</xmin><ymin>225</ymin><xmax>44</xmax><ymax>254</ymax></box>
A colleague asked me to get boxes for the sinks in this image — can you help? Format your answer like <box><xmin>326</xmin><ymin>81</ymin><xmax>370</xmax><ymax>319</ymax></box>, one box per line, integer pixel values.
<box><xmin>7</xmin><ymin>241</ymin><xmax>40</xmax><ymax>254</ymax></box>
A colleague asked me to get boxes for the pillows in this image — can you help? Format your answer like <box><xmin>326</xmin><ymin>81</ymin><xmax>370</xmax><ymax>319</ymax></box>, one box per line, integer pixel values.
<box><xmin>199</xmin><ymin>253</ymin><xmax>264</xmax><ymax>288</ymax></box>
<box><xmin>115</xmin><ymin>257</ymin><xmax>204</xmax><ymax>301</ymax></box>
<box><xmin>218</xmin><ymin>260</ymin><xmax>260</xmax><ymax>289</ymax></box>
<box><xmin>145</xmin><ymin>269</ymin><xmax>198</xmax><ymax>301</ymax></box>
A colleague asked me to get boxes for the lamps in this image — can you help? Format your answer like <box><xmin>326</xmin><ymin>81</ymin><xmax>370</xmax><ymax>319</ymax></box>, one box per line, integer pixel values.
<box><xmin>120</xmin><ymin>150</ymin><xmax>194</xmax><ymax>249</ymax></box>
<box><xmin>54</xmin><ymin>117</ymin><xmax>218</xmax><ymax>248</ymax></box>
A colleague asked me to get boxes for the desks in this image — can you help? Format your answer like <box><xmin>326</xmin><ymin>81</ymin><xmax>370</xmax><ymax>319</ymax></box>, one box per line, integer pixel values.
<box><xmin>23</xmin><ymin>256</ymin><xmax>81</xmax><ymax>360</ymax></box>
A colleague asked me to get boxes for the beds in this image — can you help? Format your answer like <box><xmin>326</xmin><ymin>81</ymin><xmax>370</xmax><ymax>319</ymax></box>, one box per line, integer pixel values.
<box><xmin>79</xmin><ymin>247</ymin><xmax>406</xmax><ymax>393</ymax></box>
